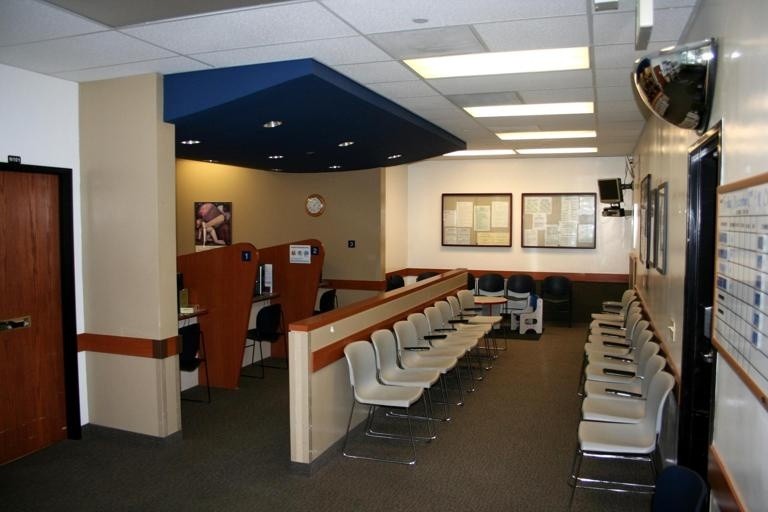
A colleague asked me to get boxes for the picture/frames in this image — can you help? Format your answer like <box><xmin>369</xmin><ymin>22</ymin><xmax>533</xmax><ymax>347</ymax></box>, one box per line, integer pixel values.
<box><xmin>521</xmin><ymin>192</ymin><xmax>597</xmax><ymax>249</ymax></box>
<box><xmin>441</xmin><ymin>193</ymin><xmax>512</xmax><ymax>247</ymax></box>
<box><xmin>639</xmin><ymin>174</ymin><xmax>668</xmax><ymax>275</ymax></box>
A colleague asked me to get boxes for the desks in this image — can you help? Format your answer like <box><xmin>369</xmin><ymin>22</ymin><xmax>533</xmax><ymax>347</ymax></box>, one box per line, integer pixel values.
<box><xmin>241</xmin><ymin>293</ymin><xmax>282</xmax><ymax>369</ymax></box>
<box><xmin>178</xmin><ymin>309</ymin><xmax>209</xmax><ymax>392</ymax></box>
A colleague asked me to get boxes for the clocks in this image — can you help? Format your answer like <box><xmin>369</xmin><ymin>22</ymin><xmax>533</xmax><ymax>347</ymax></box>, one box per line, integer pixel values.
<box><xmin>304</xmin><ymin>194</ymin><xmax>326</xmax><ymax>218</ymax></box>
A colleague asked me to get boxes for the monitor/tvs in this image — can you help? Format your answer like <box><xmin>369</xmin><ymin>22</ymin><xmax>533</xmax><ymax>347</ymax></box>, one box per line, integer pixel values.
<box><xmin>598</xmin><ymin>178</ymin><xmax>623</xmax><ymax>202</ymax></box>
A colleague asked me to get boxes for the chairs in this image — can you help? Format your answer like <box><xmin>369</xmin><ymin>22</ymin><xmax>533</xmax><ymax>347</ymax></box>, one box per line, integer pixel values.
<box><xmin>468</xmin><ymin>273</ymin><xmax>572</xmax><ymax>335</ymax></box>
<box><xmin>368</xmin><ymin>328</ymin><xmax>443</xmax><ymax>439</ymax></box>
<box><xmin>577</xmin><ymin>287</ymin><xmax>654</xmax><ymax>364</ymax></box>
<box><xmin>383</xmin><ymin>273</ymin><xmax>406</xmax><ymax>292</ymax></box>
<box><xmin>343</xmin><ymin>340</ymin><xmax>437</xmax><ymax>465</ymax></box>
<box><xmin>417</xmin><ymin>274</ymin><xmax>441</xmax><ymax>283</ymax></box>
<box><xmin>407</xmin><ymin>289</ymin><xmax>507</xmax><ymax>405</ymax></box>
<box><xmin>390</xmin><ymin>319</ymin><xmax>463</xmax><ymax>421</ymax></box>
<box><xmin>178</xmin><ymin>323</ymin><xmax>211</xmax><ymax>403</ymax></box>
<box><xmin>571</xmin><ymin>355</ymin><xmax>667</xmax><ymax>488</ymax></box>
<box><xmin>240</xmin><ymin>304</ymin><xmax>289</xmax><ymax>379</ymax></box>
<box><xmin>313</xmin><ymin>289</ymin><xmax>338</xmax><ymax>315</ymax></box>
<box><xmin>583</xmin><ymin>342</ymin><xmax>660</xmax><ymax>383</ymax></box>
<box><xmin>565</xmin><ymin>371</ymin><xmax>677</xmax><ymax>510</ymax></box>
